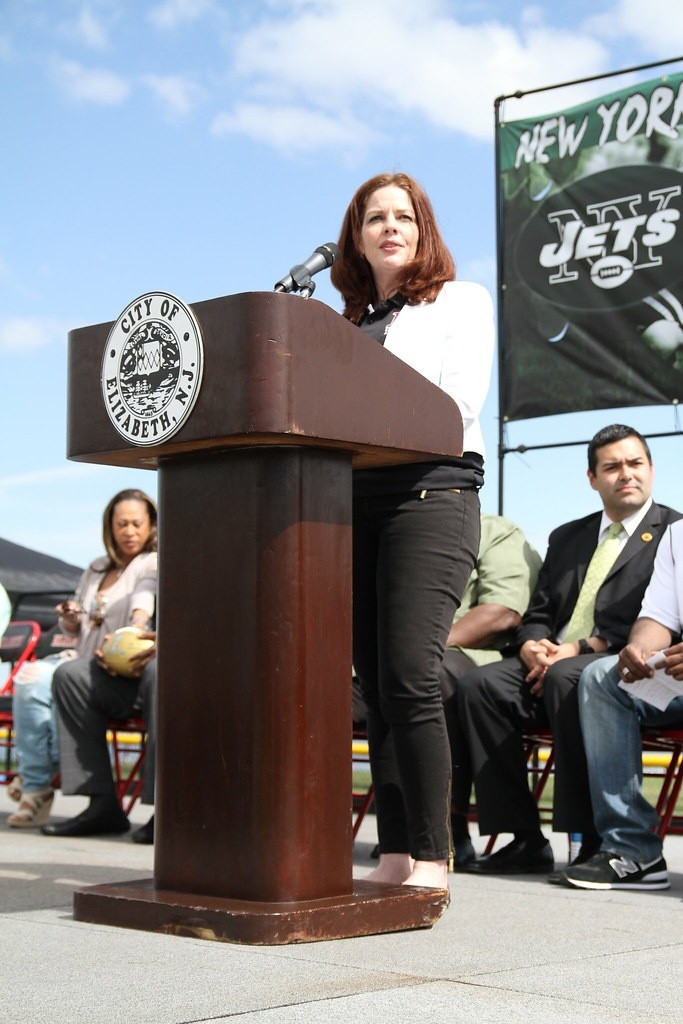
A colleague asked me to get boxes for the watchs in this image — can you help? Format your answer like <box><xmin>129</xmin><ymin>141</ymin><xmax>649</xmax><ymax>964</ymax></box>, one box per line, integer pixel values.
<box><xmin>577</xmin><ymin>638</ymin><xmax>596</xmax><ymax>655</ymax></box>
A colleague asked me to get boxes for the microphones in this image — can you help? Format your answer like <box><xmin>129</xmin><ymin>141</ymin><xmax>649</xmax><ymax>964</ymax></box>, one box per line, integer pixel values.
<box><xmin>274</xmin><ymin>243</ymin><xmax>341</xmax><ymax>294</ymax></box>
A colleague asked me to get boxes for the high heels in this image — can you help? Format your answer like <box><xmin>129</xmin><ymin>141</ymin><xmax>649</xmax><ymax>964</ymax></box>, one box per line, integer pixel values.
<box><xmin>6</xmin><ymin>776</ymin><xmax>55</xmax><ymax>828</ymax></box>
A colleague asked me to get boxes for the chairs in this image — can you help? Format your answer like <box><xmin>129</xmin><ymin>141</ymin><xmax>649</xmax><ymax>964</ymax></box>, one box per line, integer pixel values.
<box><xmin>0</xmin><ymin>622</ymin><xmax>683</xmax><ymax>856</ymax></box>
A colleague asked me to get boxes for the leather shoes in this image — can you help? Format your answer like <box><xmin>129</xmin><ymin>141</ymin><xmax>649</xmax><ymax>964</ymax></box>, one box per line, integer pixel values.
<box><xmin>469</xmin><ymin>837</ymin><xmax>556</xmax><ymax>875</ymax></box>
<box><xmin>548</xmin><ymin>842</ymin><xmax>596</xmax><ymax>884</ymax></box>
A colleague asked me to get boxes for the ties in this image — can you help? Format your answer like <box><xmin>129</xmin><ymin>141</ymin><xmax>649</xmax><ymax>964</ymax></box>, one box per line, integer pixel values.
<box><xmin>562</xmin><ymin>521</ymin><xmax>623</xmax><ymax>646</ymax></box>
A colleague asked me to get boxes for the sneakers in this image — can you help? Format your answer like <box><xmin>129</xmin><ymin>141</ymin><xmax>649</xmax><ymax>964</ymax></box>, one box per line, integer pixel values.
<box><xmin>560</xmin><ymin>850</ymin><xmax>671</xmax><ymax>890</ymax></box>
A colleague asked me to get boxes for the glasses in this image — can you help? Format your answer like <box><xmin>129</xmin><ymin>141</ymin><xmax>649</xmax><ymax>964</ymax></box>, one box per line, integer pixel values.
<box><xmin>62</xmin><ymin>592</ymin><xmax>88</xmax><ymax>615</ymax></box>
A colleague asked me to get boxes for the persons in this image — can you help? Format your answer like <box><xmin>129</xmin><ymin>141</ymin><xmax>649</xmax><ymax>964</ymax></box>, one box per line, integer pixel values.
<box><xmin>330</xmin><ymin>172</ymin><xmax>498</xmax><ymax>920</ymax></box>
<box><xmin>0</xmin><ymin>420</ymin><xmax>683</xmax><ymax>890</ymax></box>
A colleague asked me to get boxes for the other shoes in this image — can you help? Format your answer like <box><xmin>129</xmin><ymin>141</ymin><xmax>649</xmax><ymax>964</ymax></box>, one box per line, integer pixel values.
<box><xmin>129</xmin><ymin>813</ymin><xmax>155</xmax><ymax>844</ymax></box>
<box><xmin>40</xmin><ymin>805</ymin><xmax>130</xmax><ymax>839</ymax></box>
<box><xmin>448</xmin><ymin>839</ymin><xmax>477</xmax><ymax>871</ymax></box>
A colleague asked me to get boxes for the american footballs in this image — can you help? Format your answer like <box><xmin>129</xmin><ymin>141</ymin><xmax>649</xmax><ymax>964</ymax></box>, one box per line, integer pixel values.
<box><xmin>98</xmin><ymin>626</ymin><xmax>154</xmax><ymax>677</ymax></box>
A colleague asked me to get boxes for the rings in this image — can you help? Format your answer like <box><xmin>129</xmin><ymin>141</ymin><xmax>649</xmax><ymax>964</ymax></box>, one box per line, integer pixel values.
<box><xmin>622</xmin><ymin>667</ymin><xmax>630</xmax><ymax>676</ymax></box>
